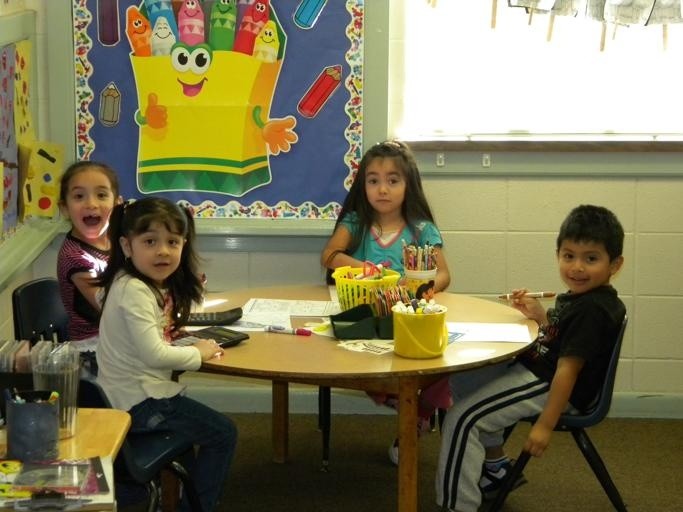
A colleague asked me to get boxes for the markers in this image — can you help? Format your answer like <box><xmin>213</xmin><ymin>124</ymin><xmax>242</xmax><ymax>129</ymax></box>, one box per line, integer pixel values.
<box><xmin>497</xmin><ymin>291</ymin><xmax>558</xmax><ymax>300</ymax></box>
<box><xmin>263</xmin><ymin>324</ymin><xmax>314</xmax><ymax>338</ymax></box>
<box><xmin>354</xmin><ymin>260</ymin><xmax>391</xmax><ymax>280</ymax></box>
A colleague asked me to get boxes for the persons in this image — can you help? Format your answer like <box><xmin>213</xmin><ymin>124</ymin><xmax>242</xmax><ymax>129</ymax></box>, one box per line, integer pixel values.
<box><xmin>55</xmin><ymin>161</ymin><xmax>123</xmax><ymax>356</ymax></box>
<box><xmin>93</xmin><ymin>196</ymin><xmax>237</xmax><ymax>512</ymax></box>
<box><xmin>320</xmin><ymin>140</ymin><xmax>452</xmax><ymax>466</ymax></box>
<box><xmin>434</xmin><ymin>205</ymin><xmax>626</xmax><ymax>511</ymax></box>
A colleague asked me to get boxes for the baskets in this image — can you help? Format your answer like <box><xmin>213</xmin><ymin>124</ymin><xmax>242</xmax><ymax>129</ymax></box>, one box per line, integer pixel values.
<box><xmin>330</xmin><ymin>265</ymin><xmax>401</xmax><ymax>312</ymax></box>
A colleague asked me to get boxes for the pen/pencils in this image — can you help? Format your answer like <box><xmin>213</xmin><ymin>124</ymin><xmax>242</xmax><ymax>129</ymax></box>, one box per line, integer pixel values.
<box><xmin>393</xmin><ymin>298</ymin><xmax>445</xmax><ymax>314</ymax></box>
<box><xmin>400</xmin><ymin>239</ymin><xmax>437</xmax><ymax>271</ymax></box>
<box><xmin>372</xmin><ymin>285</ymin><xmax>412</xmax><ymax>315</ymax></box>
<box><xmin>3</xmin><ymin>386</ymin><xmax>59</xmax><ymax>404</ymax></box>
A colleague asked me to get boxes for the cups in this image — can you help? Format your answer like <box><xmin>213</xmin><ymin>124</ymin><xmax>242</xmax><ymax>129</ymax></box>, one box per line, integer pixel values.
<box><xmin>30</xmin><ymin>342</ymin><xmax>78</xmax><ymax>440</ymax></box>
<box><xmin>402</xmin><ymin>266</ymin><xmax>437</xmax><ymax>303</ymax></box>
<box><xmin>4</xmin><ymin>391</ymin><xmax>59</xmax><ymax>462</ymax></box>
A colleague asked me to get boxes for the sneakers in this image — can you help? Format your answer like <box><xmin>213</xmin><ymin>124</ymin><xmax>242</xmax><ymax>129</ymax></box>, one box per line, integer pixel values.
<box><xmin>476</xmin><ymin>458</ymin><xmax>529</xmax><ymax>501</ymax></box>
<box><xmin>388</xmin><ymin>438</ymin><xmax>400</xmax><ymax>465</ymax></box>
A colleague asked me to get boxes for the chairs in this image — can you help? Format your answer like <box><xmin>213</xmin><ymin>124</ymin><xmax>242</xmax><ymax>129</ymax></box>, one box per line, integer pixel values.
<box><xmin>68</xmin><ymin>376</ymin><xmax>201</xmax><ymax>510</ymax></box>
<box><xmin>11</xmin><ymin>277</ymin><xmax>108</xmax><ymax>408</ymax></box>
<box><xmin>493</xmin><ymin>311</ymin><xmax>630</xmax><ymax>511</ymax></box>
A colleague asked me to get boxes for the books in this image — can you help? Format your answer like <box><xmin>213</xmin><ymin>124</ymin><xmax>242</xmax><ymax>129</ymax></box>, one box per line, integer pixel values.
<box><xmin>0</xmin><ymin>455</ymin><xmax>116</xmax><ymax>512</ymax></box>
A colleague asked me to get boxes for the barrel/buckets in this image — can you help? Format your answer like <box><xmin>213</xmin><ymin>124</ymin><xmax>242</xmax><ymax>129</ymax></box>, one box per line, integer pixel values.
<box><xmin>391</xmin><ymin>304</ymin><xmax>448</xmax><ymax>359</ymax></box>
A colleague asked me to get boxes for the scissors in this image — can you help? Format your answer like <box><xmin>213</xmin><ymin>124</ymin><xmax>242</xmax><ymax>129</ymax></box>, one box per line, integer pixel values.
<box><xmin>363</xmin><ymin>261</ymin><xmax>381</xmax><ymax>276</ymax></box>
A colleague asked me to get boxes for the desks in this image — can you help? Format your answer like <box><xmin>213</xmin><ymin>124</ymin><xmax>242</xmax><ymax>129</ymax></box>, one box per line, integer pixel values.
<box><xmin>164</xmin><ymin>283</ymin><xmax>540</xmax><ymax>511</ymax></box>
<box><xmin>0</xmin><ymin>403</ymin><xmax>132</xmax><ymax>511</ymax></box>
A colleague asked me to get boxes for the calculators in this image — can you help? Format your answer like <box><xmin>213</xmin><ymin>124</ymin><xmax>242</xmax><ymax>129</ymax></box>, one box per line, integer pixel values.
<box><xmin>178</xmin><ymin>306</ymin><xmax>245</xmax><ymax>324</ymax></box>
<box><xmin>171</xmin><ymin>325</ymin><xmax>250</xmax><ymax>359</ymax></box>
